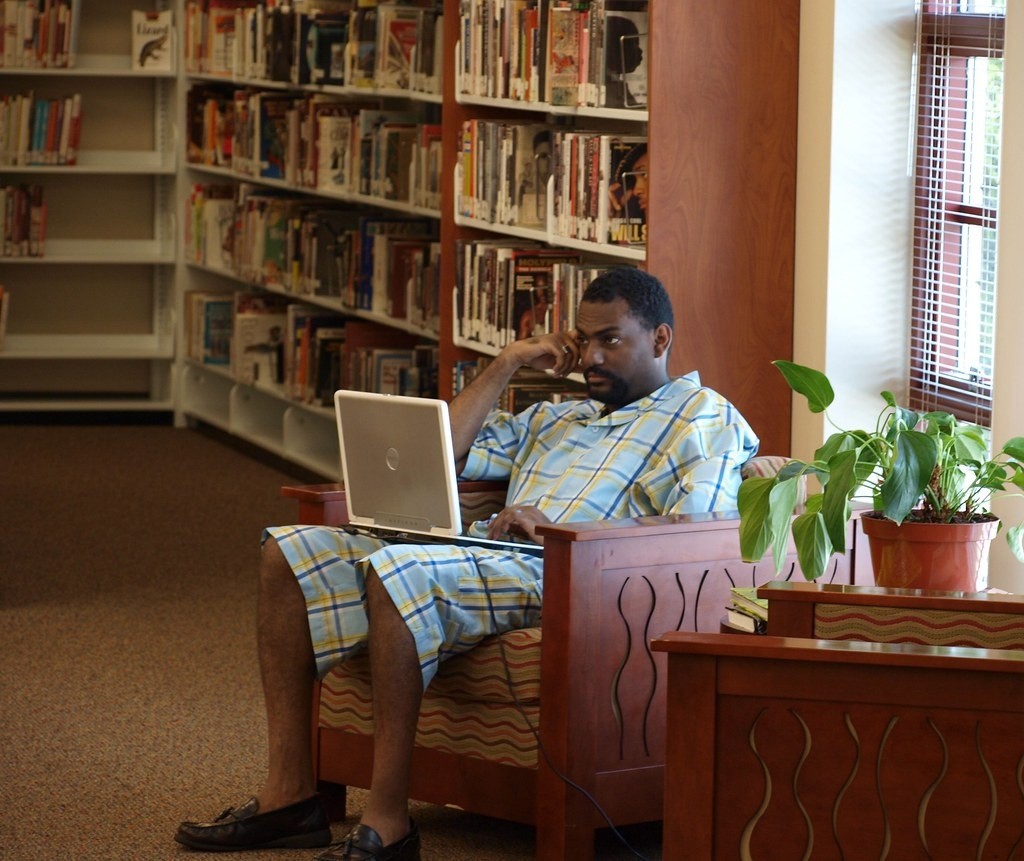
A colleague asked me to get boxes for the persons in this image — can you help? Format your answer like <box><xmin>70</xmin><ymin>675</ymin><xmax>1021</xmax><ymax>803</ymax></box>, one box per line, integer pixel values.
<box><xmin>608</xmin><ymin>146</ymin><xmax>648</xmax><ymax>235</ymax></box>
<box><xmin>519</xmin><ymin>276</ymin><xmax>549</xmax><ymax>340</ymax></box>
<box><xmin>519</xmin><ymin>130</ymin><xmax>552</xmax><ymax>205</ymax></box>
<box><xmin>174</xmin><ymin>266</ymin><xmax>761</xmax><ymax>858</ymax></box>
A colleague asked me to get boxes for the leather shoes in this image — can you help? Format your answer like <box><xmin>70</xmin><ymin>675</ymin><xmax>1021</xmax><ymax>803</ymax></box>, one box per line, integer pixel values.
<box><xmin>313</xmin><ymin>815</ymin><xmax>421</xmax><ymax>861</ymax></box>
<box><xmin>174</xmin><ymin>793</ymin><xmax>333</xmax><ymax>852</ymax></box>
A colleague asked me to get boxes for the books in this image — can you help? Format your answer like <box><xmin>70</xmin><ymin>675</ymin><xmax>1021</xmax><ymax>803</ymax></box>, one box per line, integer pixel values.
<box><xmin>0</xmin><ymin>0</ymin><xmax>81</xmax><ymax>68</ymax></box>
<box><xmin>453</xmin><ymin>237</ymin><xmax>646</xmax><ymax>369</ymax></box>
<box><xmin>183</xmin><ymin>0</ymin><xmax>442</xmax><ymax>94</ymax></box>
<box><xmin>184</xmin><ymin>290</ymin><xmax>440</xmax><ymax>408</ymax></box>
<box><xmin>453</xmin><ymin>119</ymin><xmax>648</xmax><ymax>247</ymax></box>
<box><xmin>453</xmin><ymin>358</ymin><xmax>589</xmax><ymax>416</ymax></box>
<box><xmin>185</xmin><ymin>181</ymin><xmax>441</xmax><ymax>334</ymax></box>
<box><xmin>132</xmin><ymin>9</ymin><xmax>173</xmax><ymax>70</ymax></box>
<box><xmin>0</xmin><ymin>184</ymin><xmax>49</xmax><ymax>257</ymax></box>
<box><xmin>455</xmin><ymin>0</ymin><xmax>649</xmax><ymax>109</ymax></box>
<box><xmin>0</xmin><ymin>285</ymin><xmax>10</xmax><ymax>348</ymax></box>
<box><xmin>184</xmin><ymin>80</ymin><xmax>442</xmax><ymax>209</ymax></box>
<box><xmin>0</xmin><ymin>90</ymin><xmax>85</xmax><ymax>166</ymax></box>
<box><xmin>725</xmin><ymin>588</ymin><xmax>768</xmax><ymax>633</ymax></box>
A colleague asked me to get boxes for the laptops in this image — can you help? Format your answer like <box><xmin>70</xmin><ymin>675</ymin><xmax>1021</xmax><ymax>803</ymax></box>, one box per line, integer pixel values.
<box><xmin>333</xmin><ymin>389</ymin><xmax>544</xmax><ymax>559</ymax></box>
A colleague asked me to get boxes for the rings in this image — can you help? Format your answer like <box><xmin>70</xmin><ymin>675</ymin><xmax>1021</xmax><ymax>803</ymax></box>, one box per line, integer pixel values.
<box><xmin>561</xmin><ymin>344</ymin><xmax>572</xmax><ymax>354</ymax></box>
<box><xmin>516</xmin><ymin>510</ymin><xmax>522</xmax><ymax>513</ymax></box>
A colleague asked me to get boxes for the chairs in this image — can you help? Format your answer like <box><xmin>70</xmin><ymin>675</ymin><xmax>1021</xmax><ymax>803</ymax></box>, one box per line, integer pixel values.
<box><xmin>650</xmin><ymin>581</ymin><xmax>1024</xmax><ymax>861</ymax></box>
<box><xmin>281</xmin><ymin>455</ymin><xmax>808</xmax><ymax>861</ymax></box>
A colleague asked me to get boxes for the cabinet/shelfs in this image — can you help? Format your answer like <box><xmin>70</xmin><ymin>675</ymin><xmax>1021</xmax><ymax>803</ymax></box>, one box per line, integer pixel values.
<box><xmin>0</xmin><ymin>0</ymin><xmax>180</xmax><ymax>428</ymax></box>
<box><xmin>180</xmin><ymin>0</ymin><xmax>801</xmax><ymax>482</ymax></box>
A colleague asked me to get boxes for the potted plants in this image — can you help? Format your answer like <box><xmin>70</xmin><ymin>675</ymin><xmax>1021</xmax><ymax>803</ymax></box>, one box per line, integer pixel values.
<box><xmin>736</xmin><ymin>360</ymin><xmax>1024</xmax><ymax>590</ymax></box>
